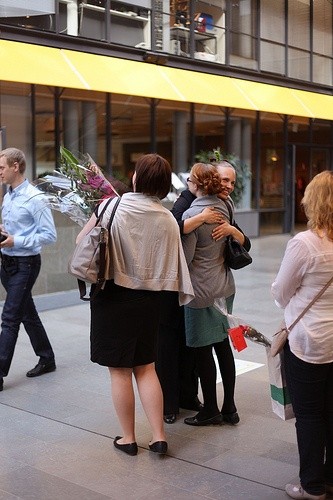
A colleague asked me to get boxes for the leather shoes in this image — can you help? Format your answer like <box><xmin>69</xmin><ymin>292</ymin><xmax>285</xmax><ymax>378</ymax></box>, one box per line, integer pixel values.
<box><xmin>26</xmin><ymin>364</ymin><xmax>55</xmax><ymax>377</ymax></box>
<box><xmin>164</xmin><ymin>414</ymin><xmax>175</xmax><ymax>423</ymax></box>
<box><xmin>194</xmin><ymin>403</ymin><xmax>204</xmax><ymax>411</ymax></box>
<box><xmin>184</xmin><ymin>413</ymin><xmax>223</xmax><ymax>426</ymax></box>
<box><xmin>224</xmin><ymin>412</ymin><xmax>239</xmax><ymax>424</ymax></box>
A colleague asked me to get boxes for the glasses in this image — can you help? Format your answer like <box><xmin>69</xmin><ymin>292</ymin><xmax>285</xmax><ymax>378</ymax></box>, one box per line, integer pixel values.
<box><xmin>187</xmin><ymin>177</ymin><xmax>193</xmax><ymax>183</ymax></box>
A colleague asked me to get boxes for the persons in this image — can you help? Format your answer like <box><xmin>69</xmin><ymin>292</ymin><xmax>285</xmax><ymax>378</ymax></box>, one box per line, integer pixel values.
<box><xmin>273</xmin><ymin>170</ymin><xmax>333</xmax><ymax>500</ymax></box>
<box><xmin>175</xmin><ymin>1</ymin><xmax>201</xmax><ymax>28</ymax></box>
<box><xmin>0</xmin><ymin>147</ymin><xmax>56</xmax><ymax>392</ymax></box>
<box><xmin>154</xmin><ymin>158</ymin><xmax>251</xmax><ymax>427</ymax></box>
<box><xmin>76</xmin><ymin>153</ymin><xmax>195</xmax><ymax>456</ymax></box>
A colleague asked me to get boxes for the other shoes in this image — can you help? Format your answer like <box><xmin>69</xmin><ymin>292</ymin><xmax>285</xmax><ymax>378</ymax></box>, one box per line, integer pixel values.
<box><xmin>148</xmin><ymin>440</ymin><xmax>167</xmax><ymax>453</ymax></box>
<box><xmin>114</xmin><ymin>435</ymin><xmax>137</xmax><ymax>456</ymax></box>
<box><xmin>323</xmin><ymin>483</ymin><xmax>333</xmax><ymax>500</ymax></box>
<box><xmin>285</xmin><ymin>484</ymin><xmax>328</xmax><ymax>500</ymax></box>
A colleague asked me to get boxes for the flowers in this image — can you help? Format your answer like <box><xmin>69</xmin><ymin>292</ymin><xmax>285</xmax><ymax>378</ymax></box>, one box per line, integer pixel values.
<box><xmin>31</xmin><ymin>145</ymin><xmax>123</xmax><ymax>228</ymax></box>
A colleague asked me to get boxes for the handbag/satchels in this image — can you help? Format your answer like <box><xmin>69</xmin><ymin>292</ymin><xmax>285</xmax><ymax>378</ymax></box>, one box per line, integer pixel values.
<box><xmin>69</xmin><ymin>197</ymin><xmax>122</xmax><ymax>300</ymax></box>
<box><xmin>269</xmin><ymin>320</ymin><xmax>288</xmax><ymax>357</ymax></box>
<box><xmin>222</xmin><ymin>200</ymin><xmax>253</xmax><ymax>270</ymax></box>
<box><xmin>265</xmin><ymin>347</ymin><xmax>295</xmax><ymax>421</ymax></box>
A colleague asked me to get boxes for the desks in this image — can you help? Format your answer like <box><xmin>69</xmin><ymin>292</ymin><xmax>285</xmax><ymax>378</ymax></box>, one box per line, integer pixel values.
<box><xmin>169</xmin><ymin>26</ymin><xmax>219</xmax><ymax>62</ymax></box>
<box><xmin>78</xmin><ymin>3</ymin><xmax>151</xmax><ymax>51</ymax></box>
